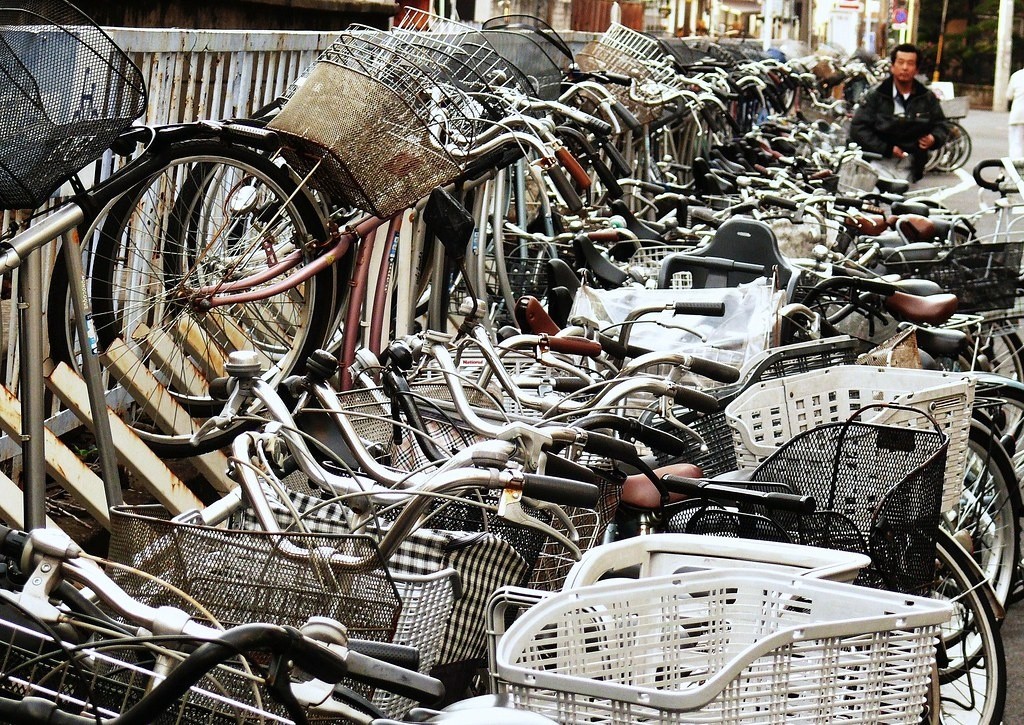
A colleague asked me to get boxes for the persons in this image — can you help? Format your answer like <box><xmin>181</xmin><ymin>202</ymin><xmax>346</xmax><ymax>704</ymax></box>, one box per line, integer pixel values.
<box><xmin>849</xmin><ymin>44</ymin><xmax>948</xmax><ymax>184</ymax></box>
<box><xmin>1005</xmin><ymin>68</ymin><xmax>1024</xmax><ymax>167</ymax></box>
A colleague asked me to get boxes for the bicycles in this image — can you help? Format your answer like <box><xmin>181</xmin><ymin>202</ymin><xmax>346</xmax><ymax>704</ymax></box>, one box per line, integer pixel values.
<box><xmin>0</xmin><ymin>0</ymin><xmax>1023</xmax><ymax>725</ymax></box>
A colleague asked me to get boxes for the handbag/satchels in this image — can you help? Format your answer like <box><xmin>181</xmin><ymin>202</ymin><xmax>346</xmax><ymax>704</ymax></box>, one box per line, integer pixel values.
<box><xmin>874</xmin><ymin>111</ymin><xmax>930</xmax><ymax>153</ymax></box>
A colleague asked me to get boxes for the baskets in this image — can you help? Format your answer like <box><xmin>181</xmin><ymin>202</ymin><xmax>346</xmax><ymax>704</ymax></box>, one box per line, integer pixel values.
<box><xmin>0</xmin><ymin>0</ymin><xmax>1024</xmax><ymax>725</ymax></box>
